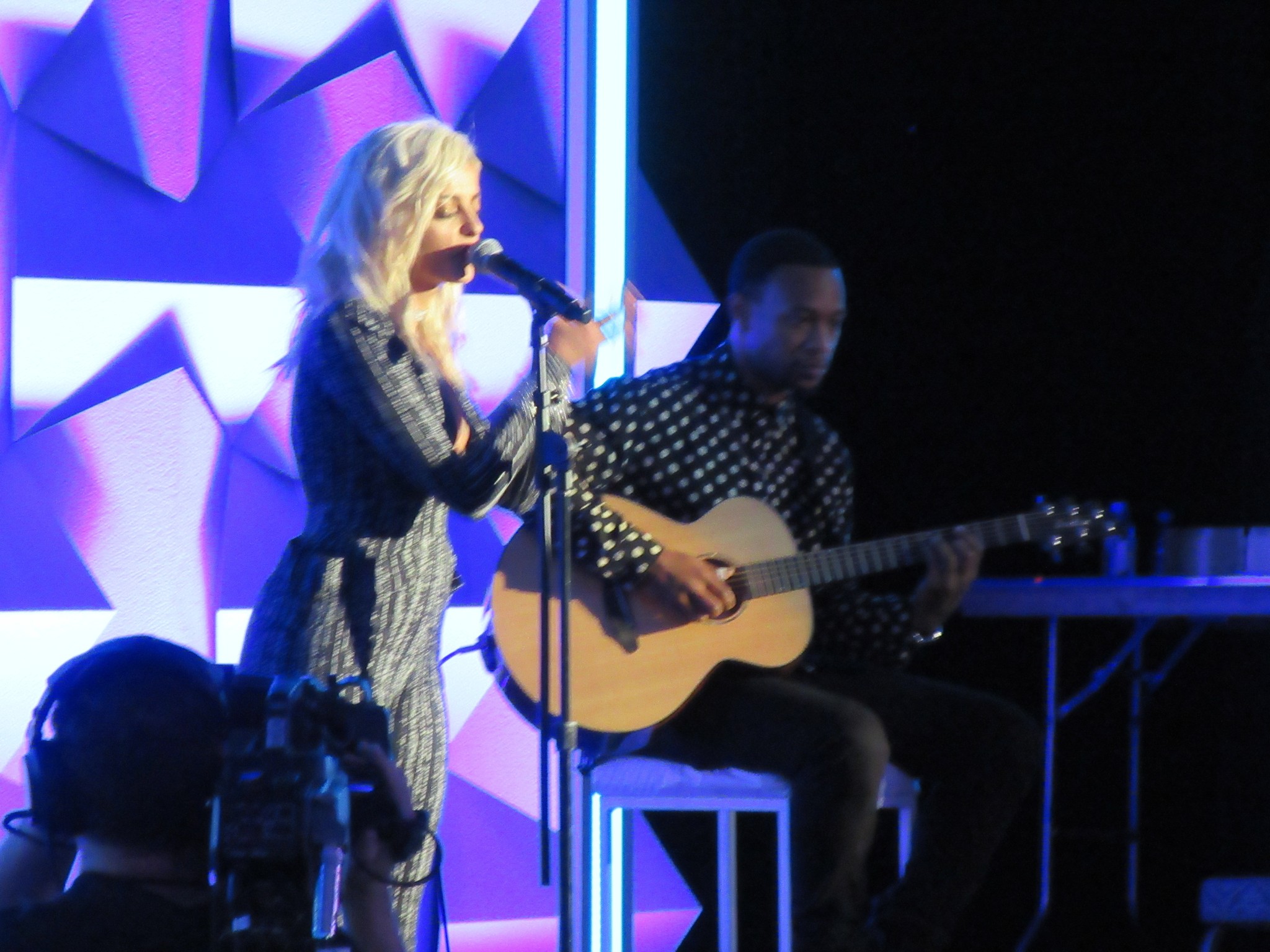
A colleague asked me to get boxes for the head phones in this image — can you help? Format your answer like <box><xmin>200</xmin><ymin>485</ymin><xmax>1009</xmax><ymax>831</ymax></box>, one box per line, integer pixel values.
<box><xmin>20</xmin><ymin>636</ymin><xmax>236</xmax><ymax>839</ymax></box>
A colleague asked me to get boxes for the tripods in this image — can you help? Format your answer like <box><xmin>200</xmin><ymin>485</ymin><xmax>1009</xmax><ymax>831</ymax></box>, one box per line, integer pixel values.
<box><xmin>524</xmin><ymin>314</ymin><xmax>584</xmax><ymax>952</ymax></box>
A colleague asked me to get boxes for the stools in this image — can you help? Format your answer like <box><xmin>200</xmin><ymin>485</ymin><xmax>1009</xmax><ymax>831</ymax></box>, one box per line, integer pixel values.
<box><xmin>594</xmin><ymin>757</ymin><xmax>927</xmax><ymax>952</ymax></box>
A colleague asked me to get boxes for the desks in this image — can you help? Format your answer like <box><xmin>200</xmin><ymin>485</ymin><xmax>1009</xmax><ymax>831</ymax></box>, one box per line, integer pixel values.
<box><xmin>956</xmin><ymin>575</ymin><xmax>1270</xmax><ymax>952</ymax></box>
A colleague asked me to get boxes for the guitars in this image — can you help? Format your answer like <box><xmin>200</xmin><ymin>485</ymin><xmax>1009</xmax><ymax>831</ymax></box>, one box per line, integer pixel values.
<box><xmin>477</xmin><ymin>493</ymin><xmax>1128</xmax><ymax>759</ymax></box>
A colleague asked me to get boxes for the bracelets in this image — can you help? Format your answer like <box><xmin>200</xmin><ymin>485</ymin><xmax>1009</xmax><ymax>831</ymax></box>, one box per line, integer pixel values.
<box><xmin>903</xmin><ymin>627</ymin><xmax>942</xmax><ymax>649</ymax></box>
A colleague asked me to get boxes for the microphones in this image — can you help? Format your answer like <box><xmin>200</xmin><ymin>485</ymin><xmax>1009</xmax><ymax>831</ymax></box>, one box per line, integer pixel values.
<box><xmin>465</xmin><ymin>236</ymin><xmax>593</xmax><ymax>329</ymax></box>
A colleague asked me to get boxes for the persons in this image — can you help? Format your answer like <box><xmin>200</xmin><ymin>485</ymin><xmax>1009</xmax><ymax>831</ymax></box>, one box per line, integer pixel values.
<box><xmin>227</xmin><ymin>111</ymin><xmax>609</xmax><ymax>951</ymax></box>
<box><xmin>514</xmin><ymin>227</ymin><xmax>986</xmax><ymax>952</ymax></box>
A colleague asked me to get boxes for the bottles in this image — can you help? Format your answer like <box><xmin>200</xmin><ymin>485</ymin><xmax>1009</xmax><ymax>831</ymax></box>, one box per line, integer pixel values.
<box><xmin>1155</xmin><ymin>511</ymin><xmax>1181</xmax><ymax>579</ymax></box>
<box><xmin>1106</xmin><ymin>507</ymin><xmax>1142</xmax><ymax>581</ymax></box>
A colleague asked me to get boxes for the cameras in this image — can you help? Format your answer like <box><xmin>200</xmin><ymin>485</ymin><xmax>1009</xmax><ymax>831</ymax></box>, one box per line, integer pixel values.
<box><xmin>204</xmin><ymin>653</ymin><xmax>431</xmax><ymax>950</ymax></box>
